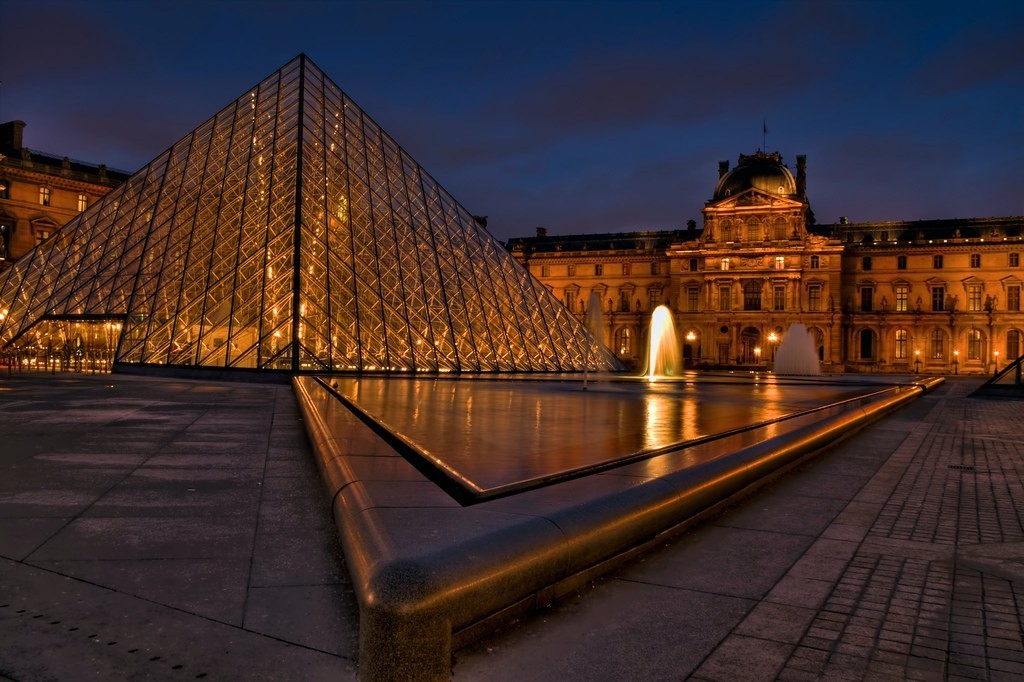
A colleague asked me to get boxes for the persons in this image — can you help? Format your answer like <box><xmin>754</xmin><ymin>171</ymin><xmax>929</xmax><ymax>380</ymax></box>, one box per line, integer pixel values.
<box><xmin>992</xmin><ymin>294</ymin><xmax>998</xmax><ymax>310</ymax></box>
<box><xmin>64</xmin><ymin>339</ymin><xmax>71</xmax><ymax>361</ymax></box>
<box><xmin>984</xmin><ymin>294</ymin><xmax>993</xmax><ymax>309</ymax></box>
<box><xmin>608</xmin><ymin>298</ymin><xmax>613</xmax><ymax>310</ymax></box>
<box><xmin>636</xmin><ymin>299</ymin><xmax>641</xmax><ymax>311</ymax></box>
<box><xmin>665</xmin><ymin>297</ymin><xmax>670</xmax><ymax>305</ymax></box>
<box><xmin>73</xmin><ymin>341</ymin><xmax>85</xmax><ymax>362</ymax></box>
<box><xmin>916</xmin><ymin>295</ymin><xmax>922</xmax><ymax>310</ymax></box>
<box><xmin>880</xmin><ymin>296</ymin><xmax>887</xmax><ymax>310</ymax></box>
<box><xmin>827</xmin><ymin>296</ymin><xmax>832</xmax><ymax>308</ymax></box>
<box><xmin>580</xmin><ymin>299</ymin><xmax>584</xmax><ymax>311</ymax></box>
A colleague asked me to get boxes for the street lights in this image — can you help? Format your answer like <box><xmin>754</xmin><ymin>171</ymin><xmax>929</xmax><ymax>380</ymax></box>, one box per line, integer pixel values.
<box><xmin>955</xmin><ymin>351</ymin><xmax>959</xmax><ymax>375</ymax></box>
<box><xmin>768</xmin><ymin>333</ymin><xmax>777</xmax><ymax>362</ymax></box>
<box><xmin>916</xmin><ymin>351</ymin><xmax>920</xmax><ymax>374</ymax></box>
<box><xmin>995</xmin><ymin>351</ymin><xmax>999</xmax><ymax>374</ymax></box>
